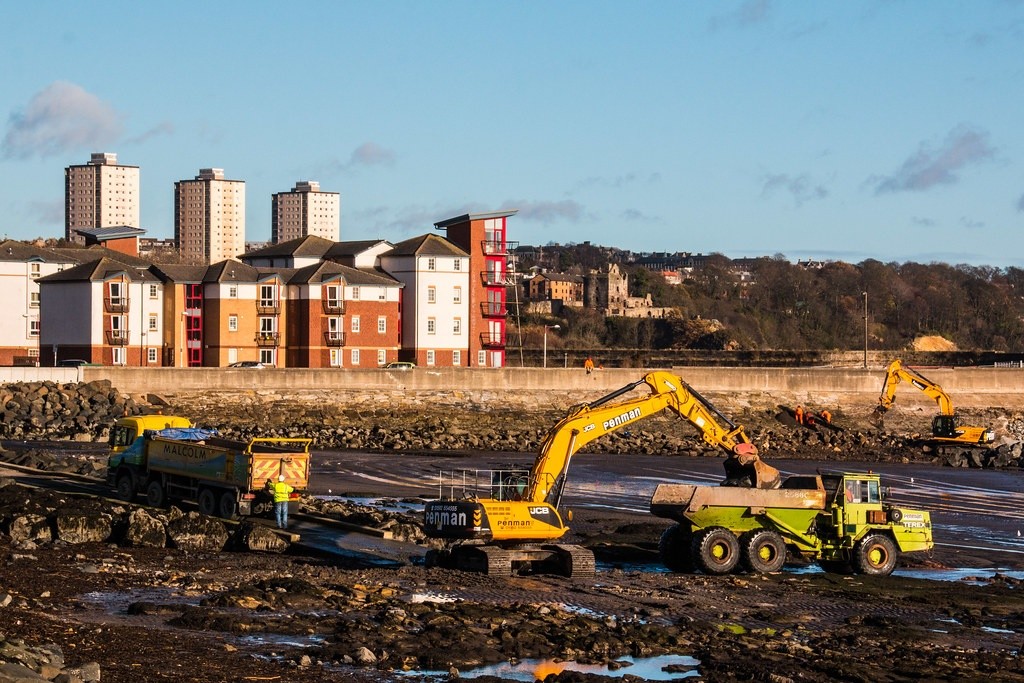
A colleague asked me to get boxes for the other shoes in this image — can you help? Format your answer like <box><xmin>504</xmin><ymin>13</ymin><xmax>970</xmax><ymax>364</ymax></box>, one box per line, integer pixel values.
<box><xmin>274</xmin><ymin>526</ymin><xmax>281</xmax><ymax>528</ymax></box>
<box><xmin>284</xmin><ymin>525</ymin><xmax>287</xmax><ymax>530</ymax></box>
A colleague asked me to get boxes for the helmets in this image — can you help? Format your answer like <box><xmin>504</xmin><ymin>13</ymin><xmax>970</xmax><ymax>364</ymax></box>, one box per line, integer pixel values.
<box><xmin>278</xmin><ymin>475</ymin><xmax>285</xmax><ymax>482</ymax></box>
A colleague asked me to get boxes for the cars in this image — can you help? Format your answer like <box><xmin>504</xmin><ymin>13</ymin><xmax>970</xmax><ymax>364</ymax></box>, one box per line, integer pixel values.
<box><xmin>226</xmin><ymin>361</ymin><xmax>265</xmax><ymax>369</ymax></box>
<box><xmin>379</xmin><ymin>362</ymin><xmax>416</xmax><ymax>368</ymax></box>
<box><xmin>55</xmin><ymin>360</ymin><xmax>87</xmax><ymax>366</ymax></box>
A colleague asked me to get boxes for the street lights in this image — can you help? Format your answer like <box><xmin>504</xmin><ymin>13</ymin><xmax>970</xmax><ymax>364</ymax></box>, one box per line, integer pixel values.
<box><xmin>544</xmin><ymin>325</ymin><xmax>562</xmax><ymax>368</ymax></box>
<box><xmin>861</xmin><ymin>291</ymin><xmax>868</xmax><ymax>367</ymax></box>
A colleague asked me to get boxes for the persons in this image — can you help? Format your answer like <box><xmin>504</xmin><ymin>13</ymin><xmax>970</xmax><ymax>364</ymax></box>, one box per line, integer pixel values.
<box><xmin>846</xmin><ymin>482</ymin><xmax>853</xmax><ymax>503</ymax></box>
<box><xmin>821</xmin><ymin>409</ymin><xmax>832</xmax><ymax>425</ymax></box>
<box><xmin>806</xmin><ymin>411</ymin><xmax>816</xmax><ymax>425</ymax></box>
<box><xmin>794</xmin><ymin>405</ymin><xmax>804</xmax><ymax>426</ymax></box>
<box><xmin>598</xmin><ymin>363</ymin><xmax>603</xmax><ymax>369</ymax></box>
<box><xmin>268</xmin><ymin>475</ymin><xmax>294</xmax><ymax>530</ymax></box>
<box><xmin>585</xmin><ymin>356</ymin><xmax>594</xmax><ymax>374</ymax></box>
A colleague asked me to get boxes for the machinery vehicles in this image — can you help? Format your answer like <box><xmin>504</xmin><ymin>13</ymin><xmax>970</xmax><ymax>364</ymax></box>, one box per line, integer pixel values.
<box><xmin>873</xmin><ymin>357</ymin><xmax>1010</xmax><ymax>458</ymax></box>
<box><xmin>423</xmin><ymin>369</ymin><xmax>784</xmax><ymax>578</ymax></box>
<box><xmin>652</xmin><ymin>469</ymin><xmax>935</xmax><ymax>579</ymax></box>
<box><xmin>106</xmin><ymin>412</ymin><xmax>312</xmax><ymax>519</ymax></box>
